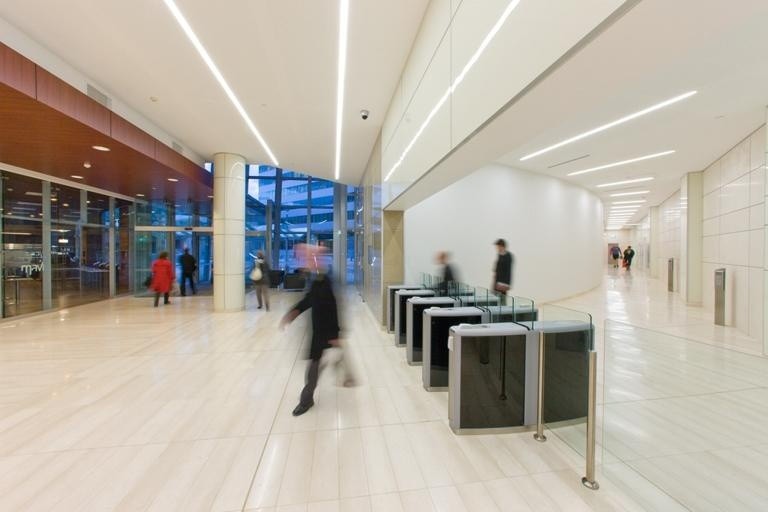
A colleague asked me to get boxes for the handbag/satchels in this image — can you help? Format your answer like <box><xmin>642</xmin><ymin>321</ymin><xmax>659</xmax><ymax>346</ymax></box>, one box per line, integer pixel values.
<box><xmin>248</xmin><ymin>267</ymin><xmax>263</xmax><ymax>282</ymax></box>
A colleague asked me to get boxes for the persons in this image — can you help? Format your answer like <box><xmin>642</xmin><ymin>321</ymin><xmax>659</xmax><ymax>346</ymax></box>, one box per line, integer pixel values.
<box><xmin>610</xmin><ymin>244</ymin><xmax>623</xmax><ymax>268</ymax></box>
<box><xmin>180</xmin><ymin>248</ymin><xmax>197</xmax><ymax>296</ymax></box>
<box><xmin>276</xmin><ymin>240</ymin><xmax>358</xmax><ymax>416</ymax></box>
<box><xmin>145</xmin><ymin>252</ymin><xmax>177</xmax><ymax>307</ymax></box>
<box><xmin>249</xmin><ymin>251</ymin><xmax>270</xmax><ymax>311</ymax></box>
<box><xmin>494</xmin><ymin>238</ymin><xmax>513</xmax><ymax>296</ymax></box>
<box><xmin>623</xmin><ymin>246</ymin><xmax>635</xmax><ymax>270</ymax></box>
<box><xmin>435</xmin><ymin>249</ymin><xmax>456</xmax><ymax>289</ymax></box>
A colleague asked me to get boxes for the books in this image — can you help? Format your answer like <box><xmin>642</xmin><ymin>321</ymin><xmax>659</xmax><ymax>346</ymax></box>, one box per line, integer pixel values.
<box><xmin>496</xmin><ymin>281</ymin><xmax>510</xmax><ymax>291</ymax></box>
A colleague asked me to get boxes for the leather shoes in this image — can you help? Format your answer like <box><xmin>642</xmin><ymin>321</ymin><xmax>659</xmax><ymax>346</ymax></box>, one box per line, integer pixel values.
<box><xmin>293</xmin><ymin>399</ymin><xmax>314</xmax><ymax>417</ymax></box>
